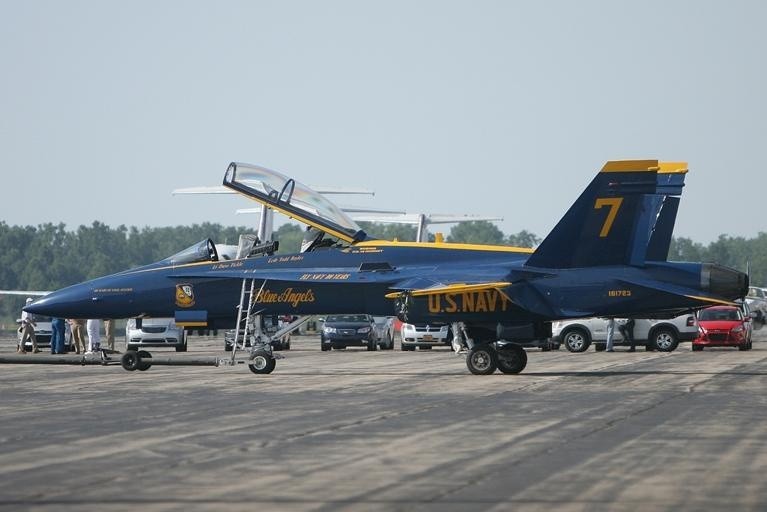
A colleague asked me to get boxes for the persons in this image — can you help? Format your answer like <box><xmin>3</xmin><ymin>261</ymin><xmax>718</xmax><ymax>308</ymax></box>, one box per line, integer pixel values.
<box><xmin>606</xmin><ymin>318</ymin><xmax>615</xmax><ymax>352</ymax></box>
<box><xmin>618</xmin><ymin>318</ymin><xmax>635</xmax><ymax>352</ymax></box>
<box><xmin>17</xmin><ymin>298</ymin><xmax>115</xmax><ymax>354</ymax></box>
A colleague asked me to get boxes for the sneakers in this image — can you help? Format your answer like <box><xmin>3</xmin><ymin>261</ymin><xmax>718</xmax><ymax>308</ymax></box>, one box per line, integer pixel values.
<box><xmin>18</xmin><ymin>349</ymin><xmax>69</xmax><ymax>354</ymax></box>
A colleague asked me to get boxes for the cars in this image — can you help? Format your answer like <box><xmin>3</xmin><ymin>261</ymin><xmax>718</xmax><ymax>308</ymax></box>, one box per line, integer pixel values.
<box><xmin>18</xmin><ymin>313</ymin><xmax>75</xmax><ymax>352</ymax></box>
<box><xmin>552</xmin><ymin>314</ymin><xmax>699</xmax><ymax>352</ymax></box>
<box><xmin>125</xmin><ymin>321</ymin><xmax>188</xmax><ymax>352</ymax></box>
<box><xmin>319</xmin><ymin>314</ymin><xmax>395</xmax><ymax>351</ymax></box>
<box><xmin>692</xmin><ymin>286</ymin><xmax>767</xmax><ymax>350</ymax></box>
<box><xmin>225</xmin><ymin>318</ymin><xmax>291</xmax><ymax>351</ymax></box>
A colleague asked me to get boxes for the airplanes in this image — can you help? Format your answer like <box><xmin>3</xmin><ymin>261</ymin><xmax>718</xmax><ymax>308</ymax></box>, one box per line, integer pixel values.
<box><xmin>21</xmin><ymin>160</ymin><xmax>749</xmax><ymax>376</ymax></box>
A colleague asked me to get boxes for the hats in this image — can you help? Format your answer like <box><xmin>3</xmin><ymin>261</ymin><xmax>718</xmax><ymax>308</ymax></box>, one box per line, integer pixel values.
<box><xmin>26</xmin><ymin>297</ymin><xmax>33</xmax><ymax>303</ymax></box>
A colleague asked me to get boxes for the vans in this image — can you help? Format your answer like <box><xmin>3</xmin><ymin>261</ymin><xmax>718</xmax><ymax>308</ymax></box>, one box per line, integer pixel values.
<box><xmin>400</xmin><ymin>323</ymin><xmax>454</xmax><ymax>350</ymax></box>
